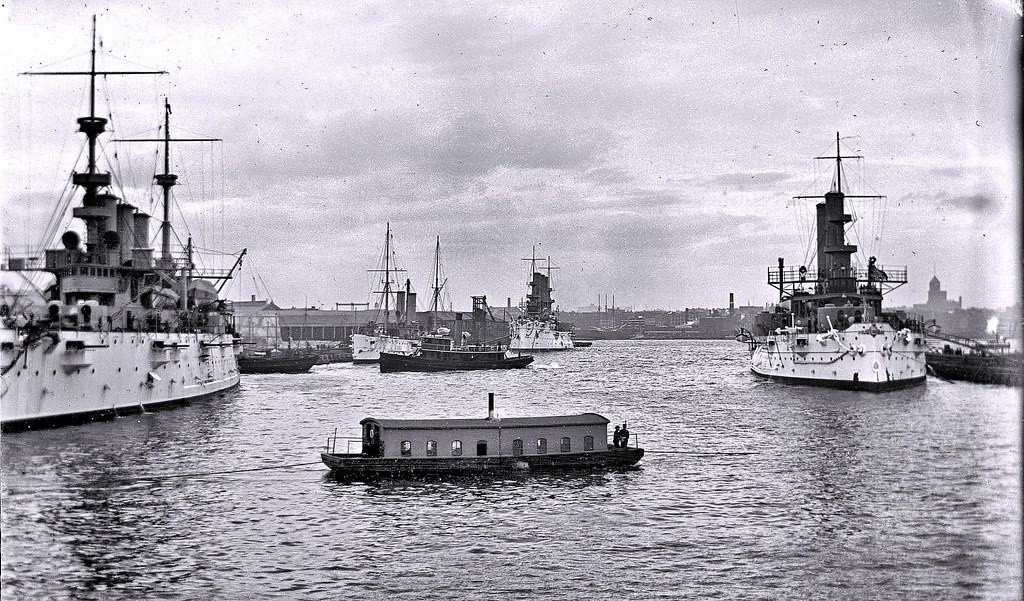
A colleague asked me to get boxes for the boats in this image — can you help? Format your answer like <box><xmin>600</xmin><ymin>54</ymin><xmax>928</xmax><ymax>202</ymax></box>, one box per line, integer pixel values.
<box><xmin>378</xmin><ymin>335</ymin><xmax>537</xmax><ymax>374</ymax></box>
<box><xmin>732</xmin><ymin>131</ymin><xmax>937</xmax><ymax>393</ymax></box>
<box><xmin>0</xmin><ymin>14</ymin><xmax>243</xmax><ymax>434</ymax></box>
<box><xmin>925</xmin><ymin>330</ymin><xmax>1024</xmax><ymax>390</ymax></box>
<box><xmin>239</xmin><ymin>346</ymin><xmax>320</xmax><ymax>374</ymax></box>
<box><xmin>318</xmin><ymin>389</ymin><xmax>646</xmax><ymax>489</ymax></box>
<box><xmin>233</xmin><ymin>297</ymin><xmax>354</xmax><ymax>366</ymax></box>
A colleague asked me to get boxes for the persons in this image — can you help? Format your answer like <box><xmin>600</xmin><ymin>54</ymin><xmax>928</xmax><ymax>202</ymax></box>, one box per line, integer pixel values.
<box><xmin>613</xmin><ymin>424</ymin><xmax>629</xmax><ymax>448</ymax></box>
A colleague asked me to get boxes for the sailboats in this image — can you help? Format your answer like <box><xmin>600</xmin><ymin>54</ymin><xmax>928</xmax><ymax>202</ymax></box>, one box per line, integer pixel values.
<box><xmin>347</xmin><ymin>222</ymin><xmax>594</xmax><ymax>367</ymax></box>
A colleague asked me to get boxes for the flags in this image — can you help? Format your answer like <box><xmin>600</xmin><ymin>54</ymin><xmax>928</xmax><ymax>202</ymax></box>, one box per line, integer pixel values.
<box><xmin>870</xmin><ymin>266</ymin><xmax>888</xmax><ymax>281</ymax></box>
